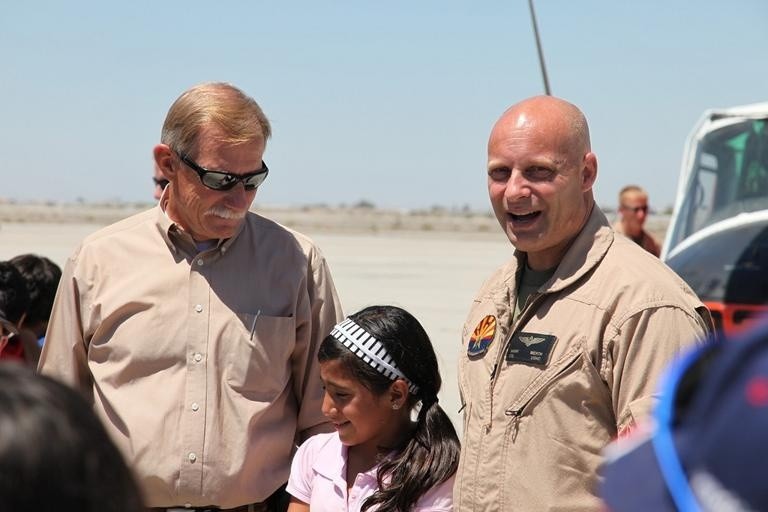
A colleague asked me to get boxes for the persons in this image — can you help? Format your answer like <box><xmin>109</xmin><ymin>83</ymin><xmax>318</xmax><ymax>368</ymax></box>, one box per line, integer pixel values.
<box><xmin>152</xmin><ymin>159</ymin><xmax>170</xmax><ymax>200</ymax></box>
<box><xmin>11</xmin><ymin>256</ymin><xmax>62</xmax><ymax>347</ymax></box>
<box><xmin>0</xmin><ymin>261</ymin><xmax>41</xmax><ymax>369</ymax></box>
<box><xmin>0</xmin><ymin>363</ymin><xmax>143</xmax><ymax>510</ymax></box>
<box><xmin>285</xmin><ymin>306</ymin><xmax>458</xmax><ymax>512</ymax></box>
<box><xmin>34</xmin><ymin>81</ymin><xmax>345</xmax><ymax>509</ymax></box>
<box><xmin>616</xmin><ymin>184</ymin><xmax>661</xmax><ymax>258</ymax></box>
<box><xmin>453</xmin><ymin>94</ymin><xmax>717</xmax><ymax>512</ymax></box>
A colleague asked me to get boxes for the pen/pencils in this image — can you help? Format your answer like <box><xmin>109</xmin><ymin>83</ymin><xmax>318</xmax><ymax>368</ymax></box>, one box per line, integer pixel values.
<box><xmin>250</xmin><ymin>310</ymin><xmax>261</xmax><ymax>341</ymax></box>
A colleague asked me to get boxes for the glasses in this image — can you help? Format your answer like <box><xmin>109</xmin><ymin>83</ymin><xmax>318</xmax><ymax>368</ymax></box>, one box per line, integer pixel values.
<box><xmin>153</xmin><ymin>177</ymin><xmax>169</xmax><ymax>190</ymax></box>
<box><xmin>624</xmin><ymin>205</ymin><xmax>648</xmax><ymax>212</ymax></box>
<box><xmin>174</xmin><ymin>149</ymin><xmax>268</xmax><ymax>192</ymax></box>
